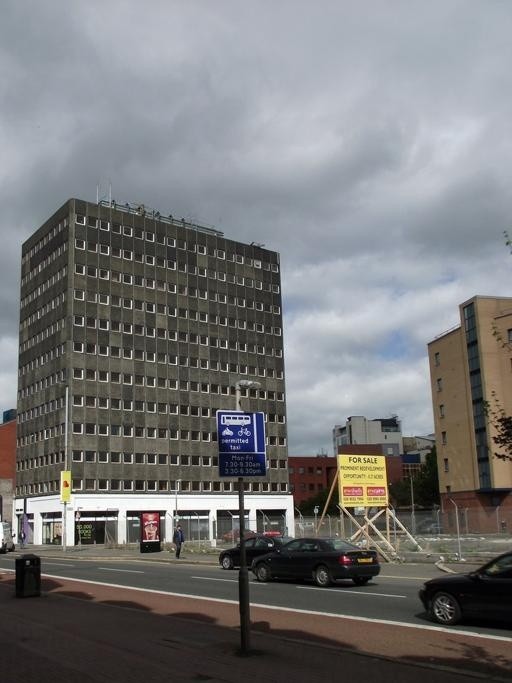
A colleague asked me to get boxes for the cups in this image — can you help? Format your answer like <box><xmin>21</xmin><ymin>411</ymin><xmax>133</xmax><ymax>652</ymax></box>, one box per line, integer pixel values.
<box><xmin>145</xmin><ymin>525</ymin><xmax>157</xmax><ymax>540</ymax></box>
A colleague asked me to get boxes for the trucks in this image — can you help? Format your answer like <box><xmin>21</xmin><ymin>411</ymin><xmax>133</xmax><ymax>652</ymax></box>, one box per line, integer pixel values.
<box><xmin>0</xmin><ymin>520</ymin><xmax>16</xmax><ymax>553</ymax></box>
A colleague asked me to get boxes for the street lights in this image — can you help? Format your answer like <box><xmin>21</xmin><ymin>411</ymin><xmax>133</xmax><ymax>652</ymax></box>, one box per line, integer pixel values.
<box><xmin>230</xmin><ymin>377</ymin><xmax>264</xmax><ymax>647</ymax></box>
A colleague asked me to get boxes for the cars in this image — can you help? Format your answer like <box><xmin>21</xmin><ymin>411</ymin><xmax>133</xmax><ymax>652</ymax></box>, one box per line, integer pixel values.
<box><xmin>417</xmin><ymin>520</ymin><xmax>444</xmax><ymax>534</ymax></box>
<box><xmin>219</xmin><ymin>531</ymin><xmax>298</xmax><ymax>569</ymax></box>
<box><xmin>301</xmin><ymin>521</ymin><xmax>315</xmax><ymax>530</ymax></box>
<box><xmin>250</xmin><ymin>535</ymin><xmax>381</xmax><ymax>586</ymax></box>
<box><xmin>223</xmin><ymin>527</ymin><xmax>255</xmax><ymax>541</ymax></box>
<box><xmin>418</xmin><ymin>549</ymin><xmax>511</xmax><ymax>632</ymax></box>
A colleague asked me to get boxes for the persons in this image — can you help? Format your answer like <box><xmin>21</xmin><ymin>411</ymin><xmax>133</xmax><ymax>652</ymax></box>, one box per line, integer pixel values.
<box><xmin>144</xmin><ymin>515</ymin><xmax>159</xmax><ymax>540</ymax></box>
<box><xmin>19</xmin><ymin>527</ymin><xmax>26</xmax><ymax>549</ymax></box>
<box><xmin>173</xmin><ymin>524</ymin><xmax>185</xmax><ymax>559</ymax></box>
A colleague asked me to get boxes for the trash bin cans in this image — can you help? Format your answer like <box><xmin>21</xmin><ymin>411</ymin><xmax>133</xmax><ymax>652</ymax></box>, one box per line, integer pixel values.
<box><xmin>14</xmin><ymin>553</ymin><xmax>42</xmax><ymax>599</ymax></box>
